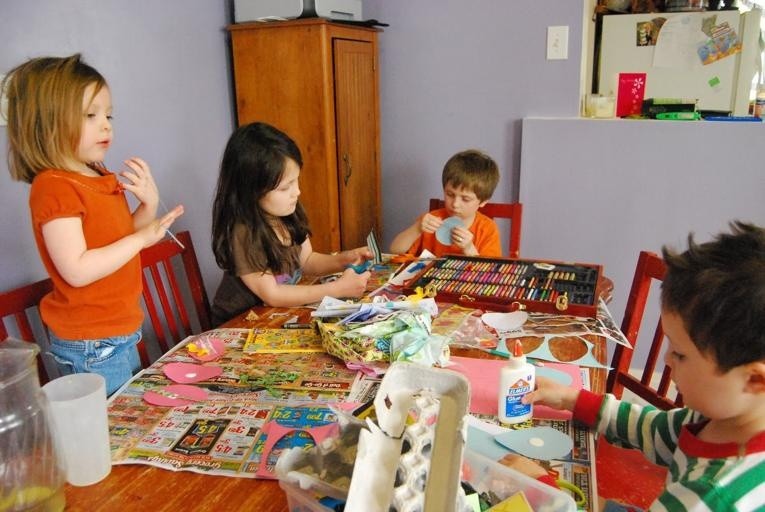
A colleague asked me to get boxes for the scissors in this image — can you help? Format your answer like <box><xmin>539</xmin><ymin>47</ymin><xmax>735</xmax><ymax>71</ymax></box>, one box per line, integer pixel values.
<box><xmin>345</xmin><ymin>260</ymin><xmax>374</xmax><ymax>273</ymax></box>
<box><xmin>390</xmin><ymin>253</ymin><xmax>447</xmax><ymax>264</ymax></box>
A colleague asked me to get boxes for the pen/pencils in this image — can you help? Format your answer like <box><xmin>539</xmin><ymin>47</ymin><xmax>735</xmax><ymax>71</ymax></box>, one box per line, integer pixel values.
<box><xmin>423</xmin><ymin>259</ymin><xmax>575</xmax><ymax>304</ymax></box>
<box><xmin>281</xmin><ymin>324</ymin><xmax>312</xmax><ymax>329</ymax></box>
<box><xmin>483</xmin><ymin>349</ymin><xmax>544</xmax><ymax>367</ymax></box>
<box><xmin>352</xmin><ymin>397</ymin><xmax>374</xmax><ymax>416</ymax></box>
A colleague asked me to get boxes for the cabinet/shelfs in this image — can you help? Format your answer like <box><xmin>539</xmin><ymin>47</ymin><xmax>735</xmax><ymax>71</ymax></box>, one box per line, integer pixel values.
<box><xmin>224</xmin><ymin>23</ymin><xmax>387</xmax><ymax>257</ymax></box>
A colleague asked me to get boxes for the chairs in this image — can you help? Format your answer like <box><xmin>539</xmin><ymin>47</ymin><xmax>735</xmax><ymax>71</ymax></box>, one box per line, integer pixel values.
<box><xmin>0</xmin><ymin>231</ymin><xmax>215</xmax><ymax>417</ymax></box>
<box><xmin>429</xmin><ymin>197</ymin><xmax>522</xmax><ymax>261</ymax></box>
<box><xmin>589</xmin><ymin>253</ymin><xmax>685</xmax><ymax>510</ymax></box>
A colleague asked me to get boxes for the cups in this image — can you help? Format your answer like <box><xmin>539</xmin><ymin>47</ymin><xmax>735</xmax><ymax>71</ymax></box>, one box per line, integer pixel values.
<box><xmin>37</xmin><ymin>373</ymin><xmax>110</xmax><ymax>487</ymax></box>
<box><xmin>584</xmin><ymin>93</ymin><xmax>614</xmax><ymax>119</ymax></box>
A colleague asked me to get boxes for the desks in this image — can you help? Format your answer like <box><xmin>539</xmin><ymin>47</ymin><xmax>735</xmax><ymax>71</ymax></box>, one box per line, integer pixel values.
<box><xmin>3</xmin><ymin>251</ymin><xmax>607</xmax><ymax>512</ymax></box>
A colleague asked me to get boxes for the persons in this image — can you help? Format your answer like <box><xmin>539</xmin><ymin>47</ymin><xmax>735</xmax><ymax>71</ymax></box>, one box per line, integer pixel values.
<box><xmin>2</xmin><ymin>54</ymin><xmax>184</xmax><ymax>399</ymax></box>
<box><xmin>521</xmin><ymin>224</ymin><xmax>764</xmax><ymax>512</ymax></box>
<box><xmin>389</xmin><ymin>150</ymin><xmax>502</xmax><ymax>260</ymax></box>
<box><xmin>211</xmin><ymin>122</ymin><xmax>376</xmax><ymax>325</ymax></box>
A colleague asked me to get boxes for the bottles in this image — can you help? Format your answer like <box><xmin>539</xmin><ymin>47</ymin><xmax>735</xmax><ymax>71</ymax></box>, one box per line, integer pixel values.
<box><xmin>1</xmin><ymin>332</ymin><xmax>69</xmax><ymax>512</ymax></box>
